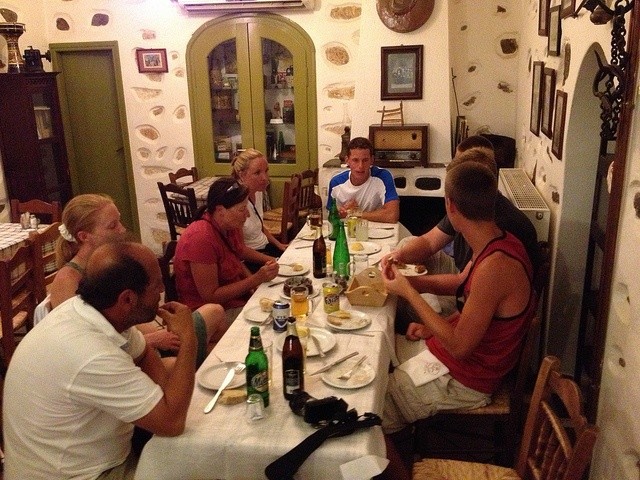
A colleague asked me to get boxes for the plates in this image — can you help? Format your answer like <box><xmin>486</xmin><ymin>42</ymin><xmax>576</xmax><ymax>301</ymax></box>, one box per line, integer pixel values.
<box><xmin>368</xmin><ymin>228</ymin><xmax>395</xmax><ymax>240</ymax></box>
<box><xmin>301</xmin><ymin>232</ymin><xmax>332</xmax><ymax>241</ymax></box>
<box><xmin>348</xmin><ymin>241</ymin><xmax>382</xmax><ymax>256</ymax></box>
<box><xmin>325</xmin><ymin>309</ymin><xmax>372</xmax><ymax>331</ymax></box>
<box><xmin>243</xmin><ymin>304</ymin><xmax>270</xmax><ymax>322</ymax></box>
<box><xmin>379</xmin><ymin>257</ymin><xmax>429</xmax><ymax>277</ymax></box>
<box><xmin>197</xmin><ymin>360</ymin><xmax>247</xmax><ymax>391</ymax></box>
<box><xmin>277</xmin><ymin>263</ymin><xmax>310</xmax><ymax>277</ymax></box>
<box><xmin>320</xmin><ymin>359</ymin><xmax>377</xmax><ymax>390</ymax></box>
<box><xmin>279</xmin><ymin>290</ymin><xmax>320</xmax><ymax>301</ymax></box>
<box><xmin>273</xmin><ymin>326</ymin><xmax>338</xmax><ymax>357</ymax></box>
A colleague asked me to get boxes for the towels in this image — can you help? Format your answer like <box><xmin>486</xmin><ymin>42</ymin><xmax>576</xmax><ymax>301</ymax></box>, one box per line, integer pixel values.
<box><xmin>397</xmin><ymin>351</ymin><xmax>449</xmax><ymax>387</ymax></box>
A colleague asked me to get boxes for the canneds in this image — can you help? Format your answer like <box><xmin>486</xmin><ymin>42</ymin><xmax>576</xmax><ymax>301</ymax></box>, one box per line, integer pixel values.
<box><xmin>273</xmin><ymin>301</ymin><xmax>290</xmax><ymax>332</ymax></box>
<box><xmin>348</xmin><ymin>217</ymin><xmax>358</xmax><ymax>237</ymax></box>
<box><xmin>323</xmin><ymin>285</ymin><xmax>339</xmax><ymax>314</ymax></box>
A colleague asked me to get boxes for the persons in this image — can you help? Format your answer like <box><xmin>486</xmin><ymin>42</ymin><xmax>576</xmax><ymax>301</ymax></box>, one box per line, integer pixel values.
<box><xmin>326</xmin><ymin>137</ymin><xmax>401</xmax><ymax>223</ymax></box>
<box><xmin>174</xmin><ymin>177</ymin><xmax>280</xmax><ymax>324</ymax></box>
<box><xmin>380</xmin><ymin>160</ymin><xmax>536</xmax><ymax>478</ymax></box>
<box><xmin>231</xmin><ymin>148</ymin><xmax>289</xmax><ymax>273</ymax></box>
<box><xmin>1</xmin><ymin>241</ymin><xmax>198</xmax><ymax>479</ymax></box>
<box><xmin>382</xmin><ymin>147</ymin><xmax>538</xmax><ymax>311</ymax></box>
<box><xmin>51</xmin><ymin>193</ymin><xmax>230</xmax><ymax>369</ymax></box>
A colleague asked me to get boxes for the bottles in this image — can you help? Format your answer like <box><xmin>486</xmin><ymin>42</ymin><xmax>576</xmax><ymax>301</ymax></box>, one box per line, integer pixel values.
<box><xmin>312</xmin><ymin>223</ymin><xmax>327</xmax><ymax>279</ymax></box>
<box><xmin>277</xmin><ymin>130</ymin><xmax>285</xmax><ymax>152</ymax></box>
<box><xmin>245</xmin><ymin>326</ymin><xmax>270</xmax><ymax>408</ymax></box>
<box><xmin>245</xmin><ymin>394</ymin><xmax>265</xmax><ymax>423</ymax></box>
<box><xmin>19</xmin><ymin>212</ymin><xmax>39</xmax><ymax>230</ymax></box>
<box><xmin>333</xmin><ymin>220</ymin><xmax>351</xmax><ymax>276</ymax></box>
<box><xmin>283</xmin><ymin>316</ymin><xmax>304</xmax><ymax>400</ymax></box>
<box><xmin>328</xmin><ymin>197</ymin><xmax>341</xmax><ymax>241</ymax></box>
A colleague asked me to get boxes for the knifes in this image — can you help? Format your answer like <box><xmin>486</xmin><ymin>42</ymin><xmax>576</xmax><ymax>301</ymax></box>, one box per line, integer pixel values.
<box><xmin>310</xmin><ymin>351</ymin><xmax>360</xmax><ymax>376</ymax></box>
<box><xmin>203</xmin><ymin>366</ymin><xmax>236</xmax><ymax>413</ymax></box>
<box><xmin>311</xmin><ymin>335</ymin><xmax>327</xmax><ymax>359</ymax></box>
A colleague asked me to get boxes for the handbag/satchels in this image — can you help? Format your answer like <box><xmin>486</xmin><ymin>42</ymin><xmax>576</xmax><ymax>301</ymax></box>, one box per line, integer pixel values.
<box><xmin>262</xmin><ymin>396</ymin><xmax>382</xmax><ymax>480</ymax></box>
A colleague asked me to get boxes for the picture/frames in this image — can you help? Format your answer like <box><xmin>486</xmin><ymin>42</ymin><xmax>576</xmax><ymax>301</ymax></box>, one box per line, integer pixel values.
<box><xmin>537</xmin><ymin>0</ymin><xmax>549</xmax><ymax>35</ymax></box>
<box><xmin>381</xmin><ymin>44</ymin><xmax>422</xmax><ymax>100</ymax></box>
<box><xmin>551</xmin><ymin>89</ymin><xmax>567</xmax><ymax>159</ymax></box>
<box><xmin>529</xmin><ymin>61</ymin><xmax>545</xmax><ymax>137</ymax></box>
<box><xmin>546</xmin><ymin>5</ymin><xmax>562</xmax><ymax>56</ymax></box>
<box><xmin>561</xmin><ymin>1</ymin><xmax>575</xmax><ymax>20</ymax></box>
<box><xmin>541</xmin><ymin>68</ymin><xmax>555</xmax><ymax>139</ymax></box>
<box><xmin>136</xmin><ymin>48</ymin><xmax>168</xmax><ymax>73</ymax></box>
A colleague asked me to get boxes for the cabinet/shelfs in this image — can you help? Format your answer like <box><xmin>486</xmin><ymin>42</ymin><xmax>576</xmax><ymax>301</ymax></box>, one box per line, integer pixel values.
<box><xmin>0</xmin><ymin>72</ymin><xmax>74</xmax><ymax>223</ymax></box>
<box><xmin>321</xmin><ymin>156</ymin><xmax>446</xmax><ymax>238</ymax></box>
<box><xmin>206</xmin><ymin>36</ymin><xmax>297</xmax><ymax>166</ymax></box>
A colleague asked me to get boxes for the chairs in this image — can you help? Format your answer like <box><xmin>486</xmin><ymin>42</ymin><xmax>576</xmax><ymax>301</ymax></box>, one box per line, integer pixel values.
<box><xmin>168</xmin><ymin>167</ymin><xmax>199</xmax><ymax>184</ymax></box>
<box><xmin>1</xmin><ymin>238</ymin><xmax>36</xmax><ymax>367</ymax></box>
<box><xmin>157</xmin><ymin>182</ymin><xmax>199</xmax><ymax>240</ymax></box>
<box><xmin>10</xmin><ymin>198</ymin><xmax>61</xmax><ymax>224</ymax></box>
<box><xmin>411</xmin><ymin>354</ymin><xmax>599</xmax><ymax>480</ymax></box>
<box><xmin>30</xmin><ymin>222</ymin><xmax>64</xmax><ymax>300</ymax></box>
<box><xmin>262</xmin><ymin>176</ymin><xmax>300</xmax><ymax>244</ymax></box>
<box><xmin>262</xmin><ymin>169</ymin><xmax>318</xmax><ymax>228</ymax></box>
<box><xmin>415</xmin><ymin>289</ymin><xmax>543</xmax><ymax>465</ymax></box>
<box><xmin>158</xmin><ymin>238</ymin><xmax>182</xmax><ymax>303</ymax></box>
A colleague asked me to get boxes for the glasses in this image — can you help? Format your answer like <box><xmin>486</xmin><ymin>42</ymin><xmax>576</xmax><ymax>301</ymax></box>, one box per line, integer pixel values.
<box><xmin>289</xmin><ymin>389</ymin><xmax>318</xmax><ymax>418</ymax></box>
<box><xmin>226</xmin><ymin>179</ymin><xmax>242</xmax><ymax>193</ymax></box>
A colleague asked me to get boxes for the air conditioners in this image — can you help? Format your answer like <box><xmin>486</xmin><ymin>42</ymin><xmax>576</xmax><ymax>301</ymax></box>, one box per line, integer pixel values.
<box><xmin>178</xmin><ymin>0</ymin><xmax>316</xmax><ymax>16</ymax></box>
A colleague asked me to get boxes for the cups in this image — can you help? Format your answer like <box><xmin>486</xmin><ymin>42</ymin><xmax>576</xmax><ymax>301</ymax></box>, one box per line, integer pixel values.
<box><xmin>350</xmin><ymin>207</ymin><xmax>362</xmax><ymax>218</ymax></box>
<box><xmin>261</xmin><ymin>338</ymin><xmax>273</xmax><ymax>388</ymax></box>
<box><xmin>355</xmin><ymin>217</ymin><xmax>369</xmax><ymax>242</ymax></box>
<box><xmin>289</xmin><ymin>285</ymin><xmax>309</xmax><ymax>321</ymax></box>
<box><xmin>265</xmin><ymin>130</ymin><xmax>275</xmax><ymax>161</ymax></box>
<box><xmin>346</xmin><ymin>254</ymin><xmax>369</xmax><ymax>278</ymax></box>
<box><xmin>306</xmin><ymin>208</ymin><xmax>324</xmax><ymax>231</ymax></box>
<box><xmin>298</xmin><ymin>336</ymin><xmax>308</xmax><ymax>376</ymax></box>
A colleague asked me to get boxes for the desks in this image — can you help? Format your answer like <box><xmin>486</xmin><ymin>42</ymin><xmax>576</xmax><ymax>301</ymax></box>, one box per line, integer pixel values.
<box><xmin>1</xmin><ymin>217</ymin><xmax>57</xmax><ymax>274</ymax></box>
<box><xmin>174</xmin><ymin>174</ymin><xmax>233</xmax><ymax>209</ymax></box>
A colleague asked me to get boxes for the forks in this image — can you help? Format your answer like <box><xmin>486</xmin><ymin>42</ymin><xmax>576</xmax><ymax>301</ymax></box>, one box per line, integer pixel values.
<box><xmin>337</xmin><ymin>355</ymin><xmax>368</xmax><ymax>381</ymax></box>
<box><xmin>325</xmin><ymin>326</ymin><xmax>375</xmax><ymax>337</ymax></box>
<box><xmin>235</xmin><ymin>362</ymin><xmax>246</xmax><ymax>375</ymax></box>
<box><xmin>266</xmin><ymin>262</ymin><xmax>297</xmax><ymax>267</ymax></box>
